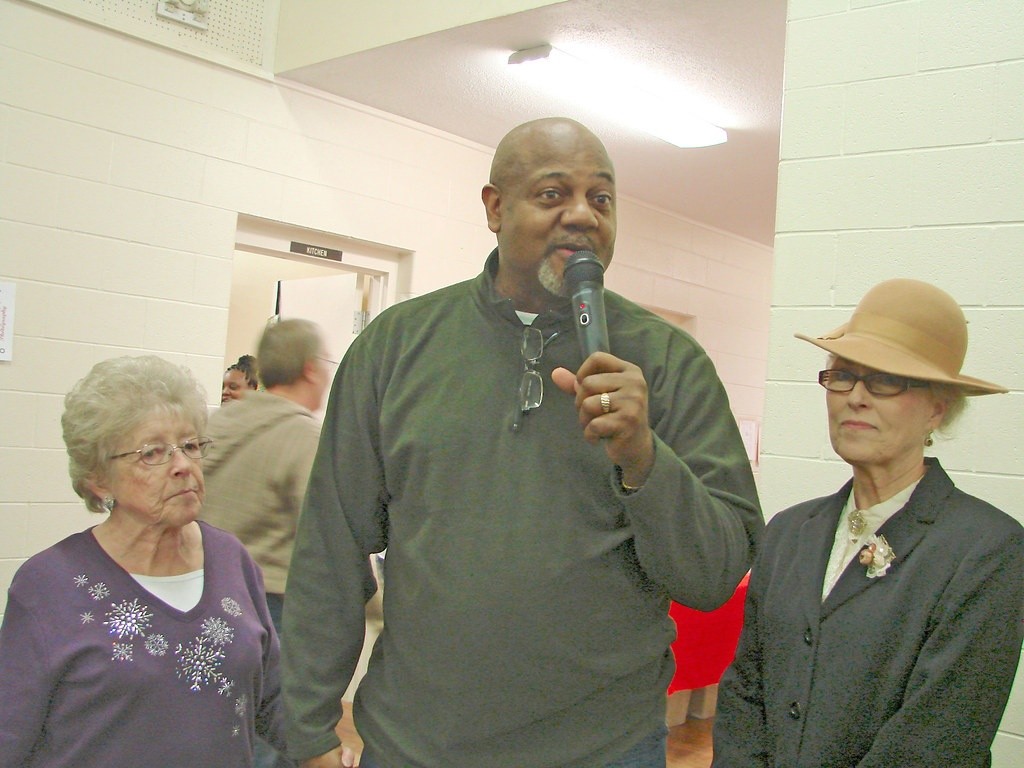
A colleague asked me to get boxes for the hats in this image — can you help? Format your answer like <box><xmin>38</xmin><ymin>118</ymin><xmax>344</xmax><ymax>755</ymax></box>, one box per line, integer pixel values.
<box><xmin>794</xmin><ymin>279</ymin><xmax>1009</xmax><ymax>396</ymax></box>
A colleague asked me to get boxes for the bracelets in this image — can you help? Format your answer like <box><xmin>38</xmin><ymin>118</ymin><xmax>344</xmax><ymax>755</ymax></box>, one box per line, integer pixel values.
<box><xmin>622</xmin><ymin>479</ymin><xmax>644</xmax><ymax>494</ymax></box>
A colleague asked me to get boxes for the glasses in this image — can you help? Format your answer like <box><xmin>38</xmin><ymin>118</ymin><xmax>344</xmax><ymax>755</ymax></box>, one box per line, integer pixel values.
<box><xmin>109</xmin><ymin>435</ymin><xmax>213</xmax><ymax>465</ymax></box>
<box><xmin>819</xmin><ymin>370</ymin><xmax>933</xmax><ymax>398</ymax></box>
<box><xmin>512</xmin><ymin>325</ymin><xmax>559</xmax><ymax>433</ymax></box>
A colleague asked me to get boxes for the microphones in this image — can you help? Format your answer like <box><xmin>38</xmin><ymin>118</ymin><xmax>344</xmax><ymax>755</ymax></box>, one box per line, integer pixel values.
<box><xmin>562</xmin><ymin>249</ymin><xmax>611</xmax><ymax>363</ymax></box>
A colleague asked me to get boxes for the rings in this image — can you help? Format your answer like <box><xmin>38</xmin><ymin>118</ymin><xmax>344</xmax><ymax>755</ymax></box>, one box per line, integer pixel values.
<box><xmin>601</xmin><ymin>392</ymin><xmax>609</xmax><ymax>413</ymax></box>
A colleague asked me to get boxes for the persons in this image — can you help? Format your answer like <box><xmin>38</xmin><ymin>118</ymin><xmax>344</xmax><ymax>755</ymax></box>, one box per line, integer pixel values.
<box><xmin>711</xmin><ymin>278</ymin><xmax>1024</xmax><ymax>768</ymax></box>
<box><xmin>201</xmin><ymin>318</ymin><xmax>332</xmax><ymax>625</ymax></box>
<box><xmin>275</xmin><ymin>116</ymin><xmax>765</xmax><ymax>768</ymax></box>
<box><xmin>0</xmin><ymin>355</ymin><xmax>354</xmax><ymax>768</ymax></box>
<box><xmin>221</xmin><ymin>354</ymin><xmax>259</xmax><ymax>406</ymax></box>
